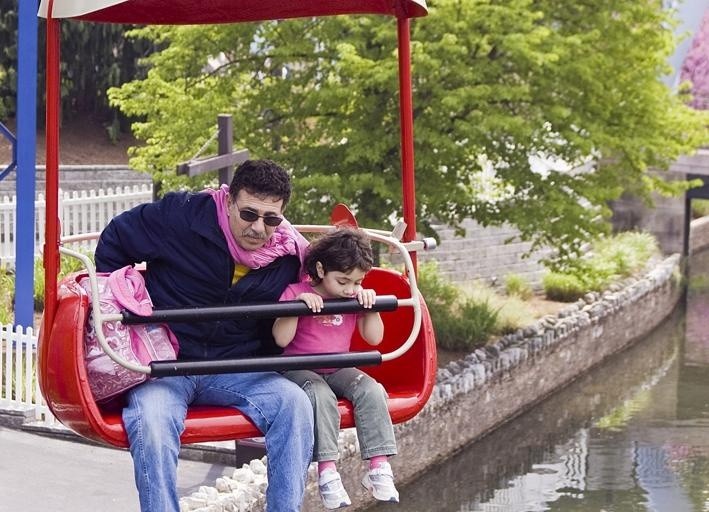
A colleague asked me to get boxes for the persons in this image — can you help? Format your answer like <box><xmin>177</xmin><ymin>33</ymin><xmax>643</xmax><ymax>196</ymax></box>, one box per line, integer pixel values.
<box><xmin>271</xmin><ymin>225</ymin><xmax>400</xmax><ymax>510</ymax></box>
<box><xmin>94</xmin><ymin>161</ymin><xmax>315</xmax><ymax>512</ymax></box>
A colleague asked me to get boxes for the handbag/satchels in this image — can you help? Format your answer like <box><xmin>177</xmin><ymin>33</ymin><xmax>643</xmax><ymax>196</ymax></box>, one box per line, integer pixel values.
<box><xmin>80</xmin><ymin>275</ymin><xmax>177</xmax><ymax>402</ymax></box>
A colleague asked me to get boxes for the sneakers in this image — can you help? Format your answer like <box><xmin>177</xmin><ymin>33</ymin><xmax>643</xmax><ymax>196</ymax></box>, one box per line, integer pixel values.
<box><xmin>318</xmin><ymin>467</ymin><xmax>352</xmax><ymax>510</ymax></box>
<box><xmin>361</xmin><ymin>462</ymin><xmax>400</xmax><ymax>503</ymax></box>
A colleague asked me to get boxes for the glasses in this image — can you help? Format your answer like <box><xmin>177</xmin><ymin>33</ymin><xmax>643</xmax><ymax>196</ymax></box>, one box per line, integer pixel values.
<box><xmin>234</xmin><ymin>196</ymin><xmax>283</xmax><ymax>226</ymax></box>
